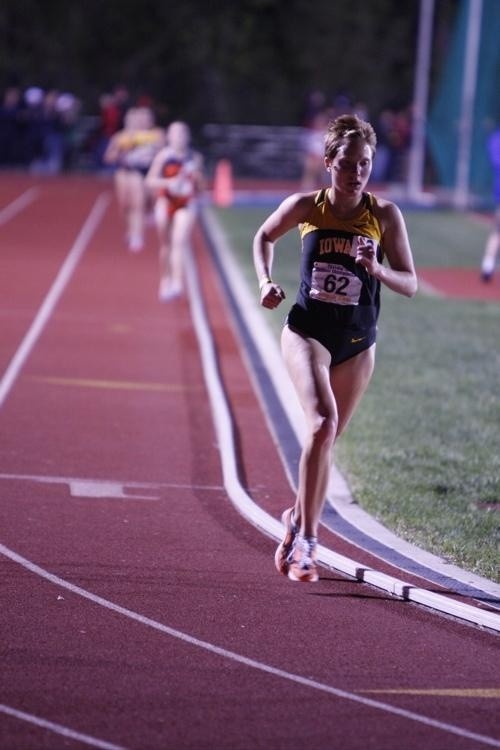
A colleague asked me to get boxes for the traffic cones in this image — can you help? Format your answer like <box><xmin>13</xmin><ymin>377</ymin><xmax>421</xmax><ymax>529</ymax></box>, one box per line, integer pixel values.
<box><xmin>211</xmin><ymin>159</ymin><xmax>232</xmax><ymax>207</ymax></box>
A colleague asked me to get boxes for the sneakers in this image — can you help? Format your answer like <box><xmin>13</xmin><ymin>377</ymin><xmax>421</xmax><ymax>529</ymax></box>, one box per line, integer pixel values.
<box><xmin>274</xmin><ymin>506</ymin><xmax>321</xmax><ymax>583</ymax></box>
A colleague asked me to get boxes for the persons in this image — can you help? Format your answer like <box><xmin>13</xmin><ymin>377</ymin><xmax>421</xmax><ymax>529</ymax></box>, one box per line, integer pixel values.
<box><xmin>253</xmin><ymin>112</ymin><xmax>420</xmax><ymax>585</ymax></box>
<box><xmin>302</xmin><ymin>90</ymin><xmax>413</xmax><ymax>194</ymax></box>
<box><xmin>146</xmin><ymin>121</ymin><xmax>204</xmax><ymax>298</ymax></box>
<box><xmin>480</xmin><ymin>130</ymin><xmax>499</xmax><ymax>281</ymax></box>
<box><xmin>0</xmin><ymin>62</ymin><xmax>164</xmax><ymax>251</ymax></box>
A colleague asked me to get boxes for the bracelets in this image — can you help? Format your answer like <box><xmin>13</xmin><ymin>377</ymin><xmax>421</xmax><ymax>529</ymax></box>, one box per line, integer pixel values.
<box><xmin>259</xmin><ymin>278</ymin><xmax>272</xmax><ymax>289</ymax></box>
